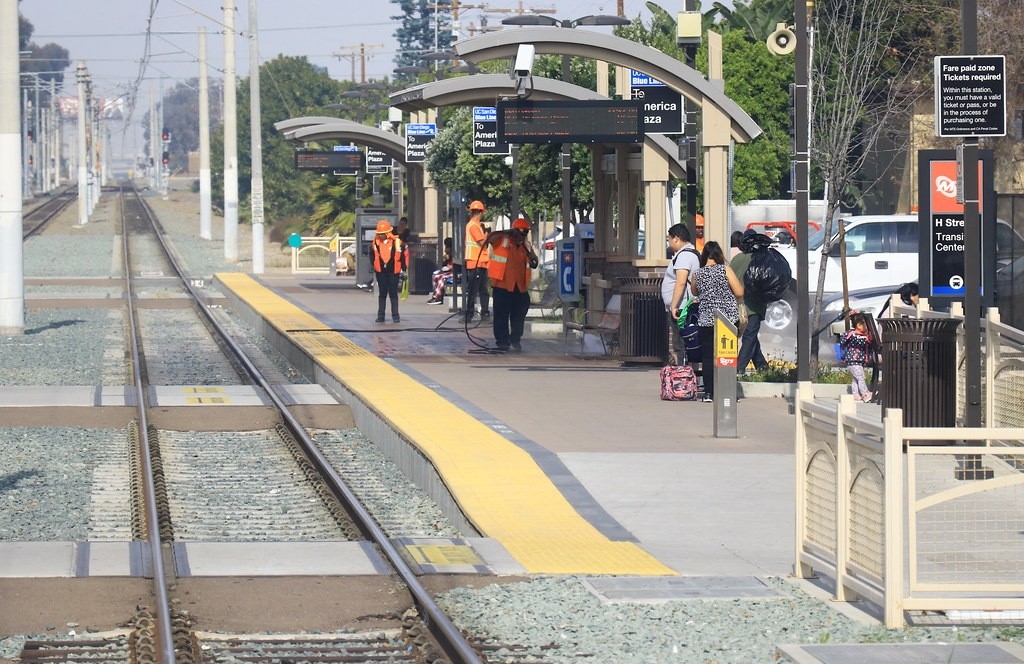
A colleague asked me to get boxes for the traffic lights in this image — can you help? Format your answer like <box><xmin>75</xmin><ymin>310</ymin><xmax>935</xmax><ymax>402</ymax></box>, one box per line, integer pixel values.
<box><xmin>162</xmin><ymin>151</ymin><xmax>169</xmax><ymax>164</ymax></box>
<box><xmin>27</xmin><ymin>132</ymin><xmax>32</xmax><ymax>141</ymax></box>
<box><xmin>162</xmin><ymin>128</ymin><xmax>169</xmax><ymax>141</ymax></box>
<box><xmin>27</xmin><ymin>155</ymin><xmax>32</xmax><ymax>163</ymax></box>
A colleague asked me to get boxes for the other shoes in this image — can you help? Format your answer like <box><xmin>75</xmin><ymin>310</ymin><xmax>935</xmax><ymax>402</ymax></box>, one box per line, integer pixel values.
<box><xmin>393</xmin><ymin>318</ymin><xmax>400</xmax><ymax>323</ymax></box>
<box><xmin>497</xmin><ymin>340</ymin><xmax>521</xmax><ymax>350</ymax></box>
<box><xmin>356</xmin><ymin>282</ymin><xmax>373</xmax><ymax>293</ymax></box>
<box><xmin>427</xmin><ymin>298</ymin><xmax>442</xmax><ymax>304</ymax></box>
<box><xmin>375</xmin><ymin>317</ymin><xmax>385</xmax><ymax>322</ymax></box>
<box><xmin>458</xmin><ymin>315</ymin><xmax>493</xmax><ymax>324</ymax></box>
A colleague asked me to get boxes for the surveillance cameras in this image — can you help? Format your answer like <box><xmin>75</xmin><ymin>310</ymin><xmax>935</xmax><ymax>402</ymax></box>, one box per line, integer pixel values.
<box><xmin>513</xmin><ymin>43</ymin><xmax>535</xmax><ymax>96</ymax></box>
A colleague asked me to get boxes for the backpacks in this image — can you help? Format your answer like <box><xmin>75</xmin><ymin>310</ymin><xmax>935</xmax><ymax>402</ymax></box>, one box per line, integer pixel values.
<box><xmin>679</xmin><ymin>303</ymin><xmax>703</xmax><ymax>363</ymax></box>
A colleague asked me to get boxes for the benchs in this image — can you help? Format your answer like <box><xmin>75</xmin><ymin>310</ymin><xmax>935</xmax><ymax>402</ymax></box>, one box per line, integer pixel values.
<box><xmin>858</xmin><ymin>311</ymin><xmax>883</xmax><ymax>386</ymax></box>
<box><xmin>563</xmin><ymin>293</ymin><xmax>624</xmax><ymax>360</ymax></box>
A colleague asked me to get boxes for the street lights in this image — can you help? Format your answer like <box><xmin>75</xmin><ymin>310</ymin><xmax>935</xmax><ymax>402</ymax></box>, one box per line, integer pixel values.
<box><xmin>498</xmin><ymin>12</ymin><xmax>633</xmax><ymax>240</ymax></box>
<box><xmin>318</xmin><ymin>47</ymin><xmax>489</xmax><ymax>319</ymax></box>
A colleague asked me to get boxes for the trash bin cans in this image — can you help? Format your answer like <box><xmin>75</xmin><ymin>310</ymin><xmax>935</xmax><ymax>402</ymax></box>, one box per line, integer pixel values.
<box><xmin>615</xmin><ymin>276</ymin><xmax>670</xmax><ymax>367</ymax></box>
<box><xmin>407</xmin><ymin>242</ymin><xmax>439</xmax><ymax>295</ymax></box>
<box><xmin>876</xmin><ymin>317</ymin><xmax>964</xmax><ymax>454</ymax></box>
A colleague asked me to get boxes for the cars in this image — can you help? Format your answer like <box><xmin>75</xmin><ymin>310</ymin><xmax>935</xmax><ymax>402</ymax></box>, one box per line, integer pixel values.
<box><xmin>786</xmin><ymin>212</ymin><xmax>1023</xmax><ymax>330</ymax></box>
<box><xmin>994</xmin><ymin>256</ymin><xmax>1023</xmax><ymax>330</ymax></box>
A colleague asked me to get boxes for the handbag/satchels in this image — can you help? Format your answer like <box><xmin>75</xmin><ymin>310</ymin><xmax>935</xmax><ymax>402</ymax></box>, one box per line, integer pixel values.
<box><xmin>660</xmin><ymin>364</ymin><xmax>698</xmax><ymax>400</ymax></box>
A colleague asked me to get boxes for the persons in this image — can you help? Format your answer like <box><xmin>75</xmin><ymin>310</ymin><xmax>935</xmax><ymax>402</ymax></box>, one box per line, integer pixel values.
<box><xmin>369</xmin><ymin>220</ymin><xmax>406</xmax><ymax>323</ymax></box>
<box><xmin>691</xmin><ymin>239</ymin><xmax>746</xmax><ymax>403</ymax></box>
<box><xmin>839</xmin><ymin>311</ymin><xmax>874</xmax><ymax>402</ymax></box>
<box><xmin>727</xmin><ymin>227</ymin><xmax>772</xmax><ymax>376</ymax></box>
<box><xmin>463</xmin><ymin>200</ymin><xmax>492</xmax><ymax>323</ymax></box>
<box><xmin>391</xmin><ymin>217</ymin><xmax>409</xmax><ymax>293</ymax></box>
<box><xmin>660</xmin><ymin>222</ymin><xmax>707</xmax><ymax>388</ymax></box>
<box><xmin>425</xmin><ymin>237</ymin><xmax>463</xmax><ymax>306</ymax></box>
<box><xmin>728</xmin><ymin>232</ymin><xmax>745</xmax><ymax>264</ymax></box>
<box><xmin>877</xmin><ymin>282</ymin><xmax>930</xmax><ymax>352</ymax></box>
<box><xmin>486</xmin><ymin>217</ymin><xmax>538</xmax><ymax>352</ymax></box>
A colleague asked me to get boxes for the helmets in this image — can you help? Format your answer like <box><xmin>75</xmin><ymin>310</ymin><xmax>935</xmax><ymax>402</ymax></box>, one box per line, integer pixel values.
<box><xmin>375</xmin><ymin>220</ymin><xmax>393</xmax><ymax>234</ymax></box>
<box><xmin>468</xmin><ymin>200</ymin><xmax>486</xmax><ymax>213</ymax></box>
<box><xmin>511</xmin><ymin>218</ymin><xmax>529</xmax><ymax>229</ymax></box>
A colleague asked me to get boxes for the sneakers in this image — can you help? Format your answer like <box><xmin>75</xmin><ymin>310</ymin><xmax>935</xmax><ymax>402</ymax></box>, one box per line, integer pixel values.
<box><xmin>703</xmin><ymin>392</ymin><xmax>713</xmax><ymax>402</ymax></box>
<box><xmin>854</xmin><ymin>391</ymin><xmax>878</xmax><ymax>403</ymax></box>
